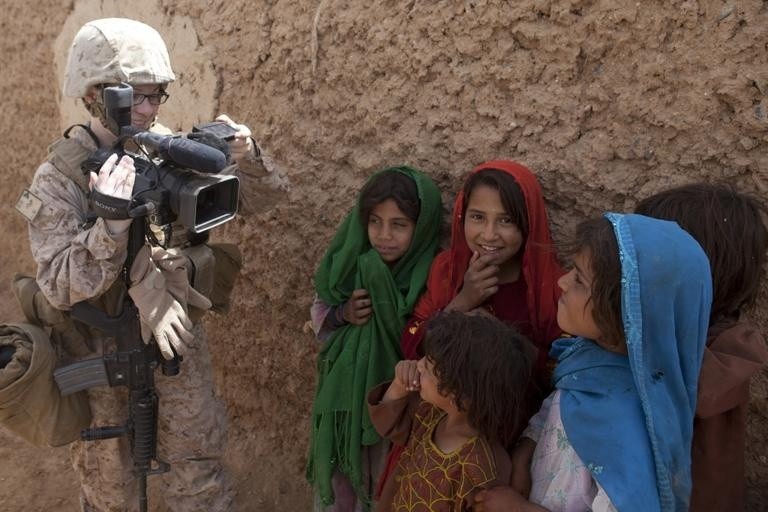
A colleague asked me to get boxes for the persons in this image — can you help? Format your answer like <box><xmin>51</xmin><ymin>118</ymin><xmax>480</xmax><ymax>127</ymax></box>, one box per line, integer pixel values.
<box><xmin>372</xmin><ymin>159</ymin><xmax>568</xmax><ymax>498</ymax></box>
<box><xmin>472</xmin><ymin>211</ymin><xmax>713</xmax><ymax>512</ymax></box>
<box><xmin>310</xmin><ymin>165</ymin><xmax>445</xmax><ymax>511</ymax></box>
<box><xmin>367</xmin><ymin>308</ymin><xmax>538</xmax><ymax>512</ymax></box>
<box><xmin>12</xmin><ymin>18</ymin><xmax>287</xmax><ymax>512</ymax></box>
<box><xmin>633</xmin><ymin>184</ymin><xmax>767</xmax><ymax>512</ymax></box>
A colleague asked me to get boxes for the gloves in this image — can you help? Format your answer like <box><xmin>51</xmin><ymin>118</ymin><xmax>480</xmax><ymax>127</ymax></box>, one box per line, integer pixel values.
<box><xmin>128</xmin><ymin>244</ymin><xmax>213</xmax><ymax>362</ymax></box>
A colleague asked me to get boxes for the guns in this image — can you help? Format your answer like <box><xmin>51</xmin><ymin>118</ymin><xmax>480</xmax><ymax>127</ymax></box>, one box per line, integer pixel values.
<box><xmin>54</xmin><ymin>200</ymin><xmax>180</xmax><ymax>512</ymax></box>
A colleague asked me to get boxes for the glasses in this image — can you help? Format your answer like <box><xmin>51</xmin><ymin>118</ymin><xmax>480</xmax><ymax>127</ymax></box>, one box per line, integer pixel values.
<box><xmin>128</xmin><ymin>87</ymin><xmax>170</xmax><ymax>107</ymax></box>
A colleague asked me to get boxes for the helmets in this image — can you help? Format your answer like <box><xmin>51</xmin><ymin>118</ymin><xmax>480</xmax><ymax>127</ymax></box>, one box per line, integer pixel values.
<box><xmin>61</xmin><ymin>15</ymin><xmax>177</xmax><ymax>100</ymax></box>
